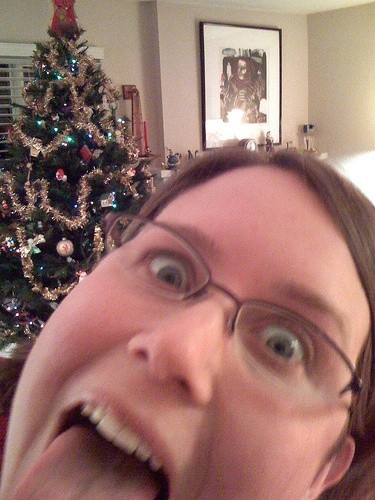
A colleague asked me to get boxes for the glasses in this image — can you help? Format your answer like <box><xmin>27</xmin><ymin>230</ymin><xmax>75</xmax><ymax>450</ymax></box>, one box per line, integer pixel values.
<box><xmin>103</xmin><ymin>210</ymin><xmax>369</xmax><ymax>403</ymax></box>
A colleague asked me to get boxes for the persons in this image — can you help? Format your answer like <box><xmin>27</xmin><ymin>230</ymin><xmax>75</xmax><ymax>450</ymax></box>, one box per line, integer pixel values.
<box><xmin>1</xmin><ymin>147</ymin><xmax>374</xmax><ymax>500</ymax></box>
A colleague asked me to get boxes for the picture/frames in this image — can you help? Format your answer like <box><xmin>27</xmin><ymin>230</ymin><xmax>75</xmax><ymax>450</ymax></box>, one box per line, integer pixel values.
<box><xmin>201</xmin><ymin>21</ymin><xmax>282</xmax><ymax>151</ymax></box>
